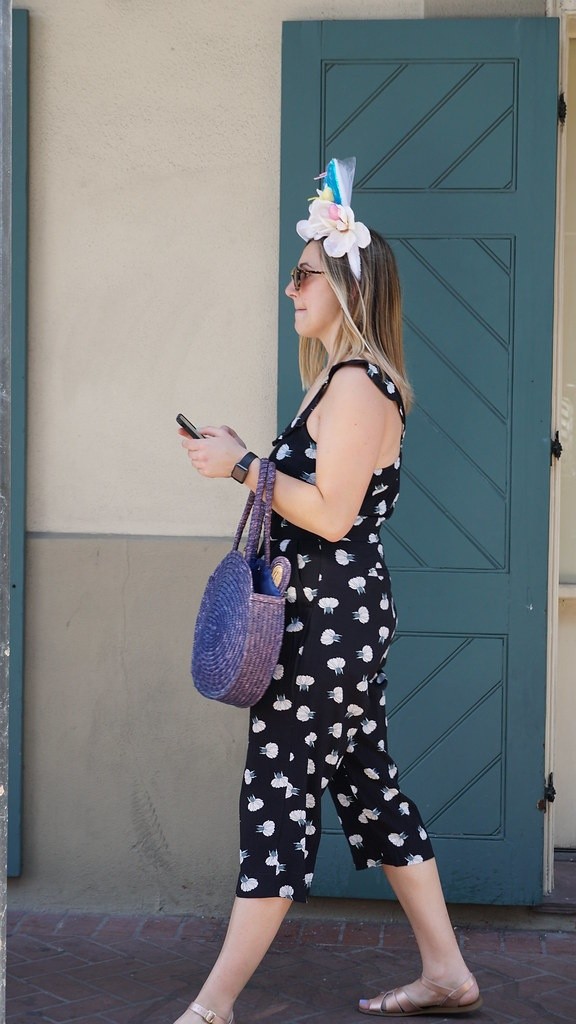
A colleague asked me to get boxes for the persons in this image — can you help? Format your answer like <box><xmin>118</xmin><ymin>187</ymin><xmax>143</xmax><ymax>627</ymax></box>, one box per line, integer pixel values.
<box><xmin>170</xmin><ymin>226</ymin><xmax>481</xmax><ymax>1024</ymax></box>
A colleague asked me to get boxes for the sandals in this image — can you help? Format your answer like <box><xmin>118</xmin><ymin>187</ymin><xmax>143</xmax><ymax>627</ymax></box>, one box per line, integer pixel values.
<box><xmin>359</xmin><ymin>972</ymin><xmax>483</xmax><ymax>1016</ymax></box>
<box><xmin>174</xmin><ymin>1001</ymin><xmax>234</xmax><ymax>1024</ymax></box>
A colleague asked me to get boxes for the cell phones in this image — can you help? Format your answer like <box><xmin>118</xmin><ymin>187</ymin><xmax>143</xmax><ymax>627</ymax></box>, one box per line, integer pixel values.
<box><xmin>176</xmin><ymin>413</ymin><xmax>205</xmax><ymax>439</ymax></box>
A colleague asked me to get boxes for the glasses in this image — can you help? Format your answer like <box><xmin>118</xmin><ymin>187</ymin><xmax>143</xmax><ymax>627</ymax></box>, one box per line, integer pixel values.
<box><xmin>290</xmin><ymin>266</ymin><xmax>325</xmax><ymax>291</ymax></box>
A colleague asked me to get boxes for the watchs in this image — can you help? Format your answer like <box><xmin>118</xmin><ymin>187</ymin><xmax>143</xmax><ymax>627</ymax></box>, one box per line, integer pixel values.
<box><xmin>230</xmin><ymin>451</ymin><xmax>259</xmax><ymax>484</ymax></box>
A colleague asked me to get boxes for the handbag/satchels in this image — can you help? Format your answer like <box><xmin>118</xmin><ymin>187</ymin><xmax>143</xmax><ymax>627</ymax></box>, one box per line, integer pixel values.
<box><xmin>190</xmin><ymin>458</ymin><xmax>291</xmax><ymax>708</ymax></box>
